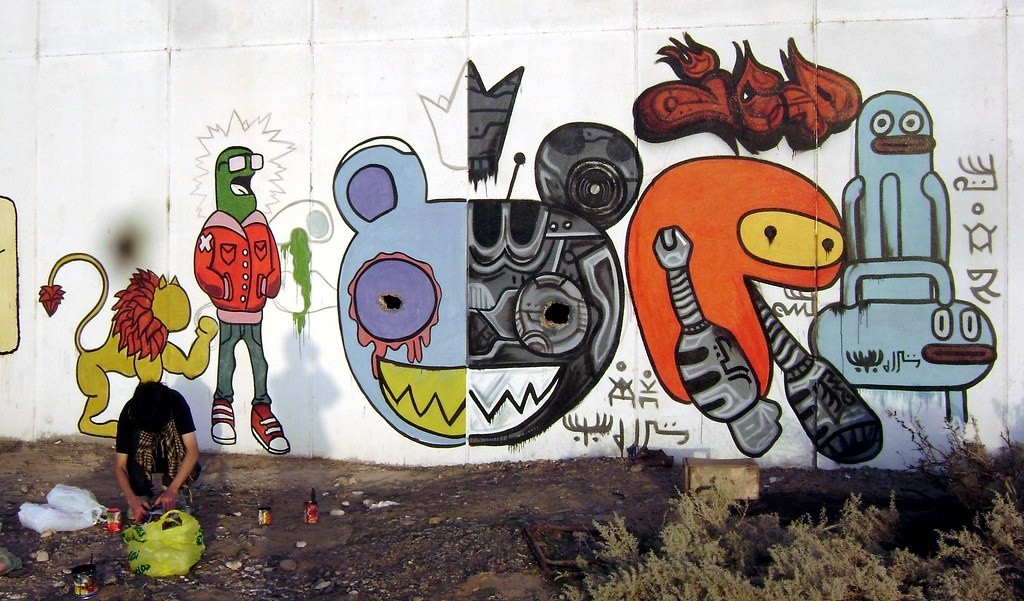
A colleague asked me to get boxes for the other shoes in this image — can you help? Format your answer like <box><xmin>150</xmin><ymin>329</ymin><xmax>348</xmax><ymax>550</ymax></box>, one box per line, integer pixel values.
<box><xmin>127</xmin><ymin>495</ymin><xmax>152</xmax><ymax>520</ymax></box>
<box><xmin>163</xmin><ymin>485</ymin><xmax>186</xmax><ymax>507</ymax></box>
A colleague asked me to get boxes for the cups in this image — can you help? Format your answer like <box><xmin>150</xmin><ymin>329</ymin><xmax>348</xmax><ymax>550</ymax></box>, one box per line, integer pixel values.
<box><xmin>72</xmin><ymin>564</ymin><xmax>99</xmax><ymax>599</ymax></box>
<box><xmin>305</xmin><ymin>502</ymin><xmax>318</xmax><ymax>523</ymax></box>
<box><xmin>108</xmin><ymin>508</ymin><xmax>121</xmax><ymax>532</ymax></box>
<box><xmin>258</xmin><ymin>506</ymin><xmax>272</xmax><ymax>527</ymax></box>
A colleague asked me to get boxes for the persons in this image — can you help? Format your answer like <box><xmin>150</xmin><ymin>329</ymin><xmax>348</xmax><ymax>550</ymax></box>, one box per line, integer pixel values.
<box><xmin>115</xmin><ymin>381</ymin><xmax>201</xmax><ymax>522</ymax></box>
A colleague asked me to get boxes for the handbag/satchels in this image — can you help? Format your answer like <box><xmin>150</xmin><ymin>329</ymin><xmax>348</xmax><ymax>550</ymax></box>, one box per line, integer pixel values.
<box><xmin>18</xmin><ymin>484</ymin><xmax>110</xmax><ymax>532</ymax></box>
<box><xmin>121</xmin><ymin>509</ymin><xmax>204</xmax><ymax>577</ymax></box>
